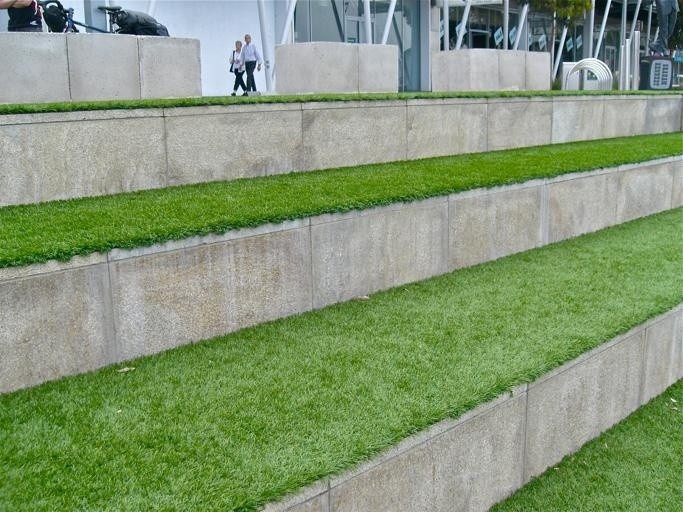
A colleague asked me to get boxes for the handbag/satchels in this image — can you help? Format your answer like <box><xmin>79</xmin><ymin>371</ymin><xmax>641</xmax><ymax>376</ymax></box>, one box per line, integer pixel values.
<box><xmin>229</xmin><ymin>64</ymin><xmax>233</xmax><ymax>72</ymax></box>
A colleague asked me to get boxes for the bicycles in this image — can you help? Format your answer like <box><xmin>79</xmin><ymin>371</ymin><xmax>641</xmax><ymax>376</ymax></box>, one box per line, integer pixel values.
<box><xmin>33</xmin><ymin>0</ymin><xmax>156</xmax><ymax>33</ymax></box>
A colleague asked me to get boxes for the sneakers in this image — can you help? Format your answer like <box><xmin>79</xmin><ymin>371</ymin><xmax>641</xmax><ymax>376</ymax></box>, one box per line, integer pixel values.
<box><xmin>231</xmin><ymin>92</ymin><xmax>248</xmax><ymax>96</ymax></box>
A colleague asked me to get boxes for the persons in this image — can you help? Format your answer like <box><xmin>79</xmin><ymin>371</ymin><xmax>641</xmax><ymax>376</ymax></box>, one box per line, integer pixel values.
<box><xmin>230</xmin><ymin>40</ymin><xmax>247</xmax><ymax>96</ymax></box>
<box><xmin>642</xmin><ymin>0</ymin><xmax>681</xmax><ymax>56</ymax></box>
<box><xmin>239</xmin><ymin>35</ymin><xmax>262</xmax><ymax>95</ymax></box>
<box><xmin>0</xmin><ymin>0</ymin><xmax>43</xmax><ymax>32</ymax></box>
